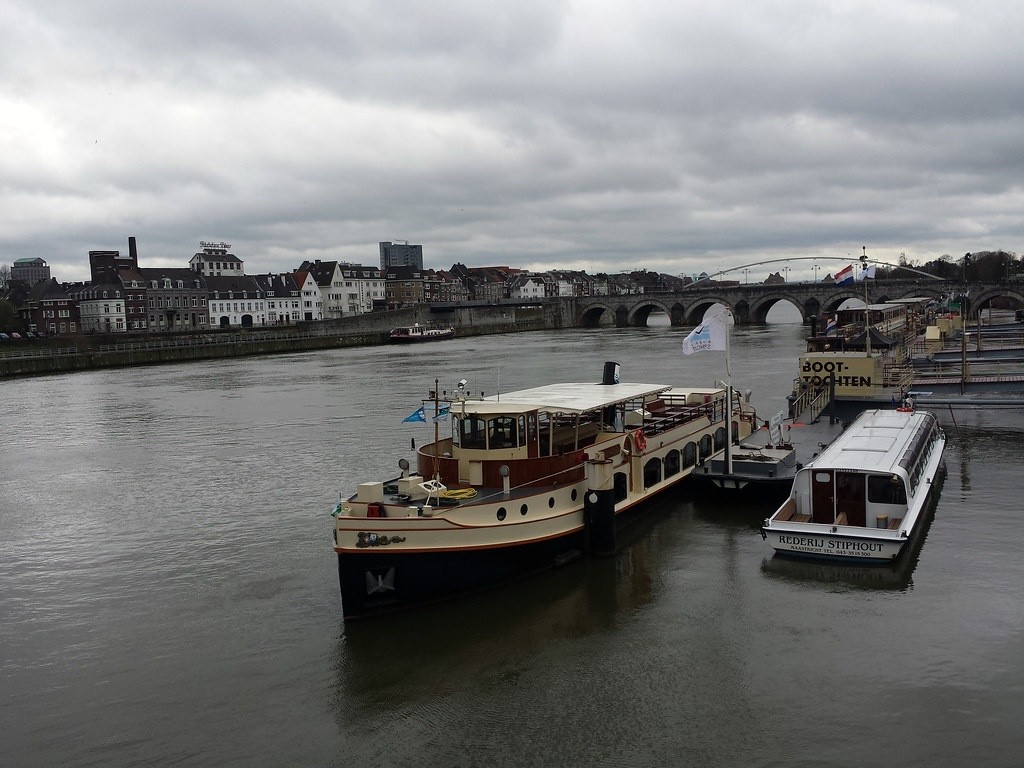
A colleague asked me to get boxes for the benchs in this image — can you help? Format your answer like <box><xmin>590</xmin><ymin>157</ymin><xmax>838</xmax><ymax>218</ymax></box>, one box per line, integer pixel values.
<box><xmin>646</xmin><ymin>398</ymin><xmax>675</xmax><ymax>416</ymax></box>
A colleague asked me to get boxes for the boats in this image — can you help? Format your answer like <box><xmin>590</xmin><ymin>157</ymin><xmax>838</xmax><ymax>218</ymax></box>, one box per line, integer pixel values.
<box><xmin>692</xmin><ymin>372</ymin><xmax>853</xmax><ymax>524</ymax></box>
<box><xmin>762</xmin><ymin>409</ymin><xmax>946</xmax><ymax>570</ymax></box>
<box><xmin>386</xmin><ymin>326</ymin><xmax>454</xmax><ymax>345</ymax></box>
<box><xmin>332</xmin><ymin>362</ymin><xmax>760</xmax><ymax>629</ymax></box>
<box><xmin>805</xmin><ymin>292</ymin><xmax>933</xmax><ymax>351</ymax></box>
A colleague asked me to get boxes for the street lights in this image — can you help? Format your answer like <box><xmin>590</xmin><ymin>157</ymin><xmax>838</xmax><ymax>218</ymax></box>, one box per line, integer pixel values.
<box><xmin>958</xmin><ymin>261</ymin><xmax>970</xmax><ymax>283</ymax></box>
<box><xmin>742</xmin><ymin>268</ymin><xmax>750</xmax><ymax>286</ymax></box>
<box><xmin>811</xmin><ymin>265</ymin><xmax>820</xmax><ymax>283</ymax></box>
<box><xmin>1002</xmin><ymin>260</ymin><xmax>1013</xmax><ymax>279</ymax></box>
<box><xmin>852</xmin><ymin>264</ymin><xmax>861</xmax><ymax>283</ymax></box>
<box><xmin>720</xmin><ymin>271</ymin><xmax>725</xmax><ymax>287</ymax></box>
<box><xmin>782</xmin><ymin>266</ymin><xmax>791</xmax><ymax>283</ymax></box>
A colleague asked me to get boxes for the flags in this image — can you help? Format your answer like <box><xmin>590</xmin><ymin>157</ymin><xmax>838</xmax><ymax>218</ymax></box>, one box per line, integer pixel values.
<box><xmin>681</xmin><ymin>309</ymin><xmax>729</xmax><ymax>355</ymax></box>
<box><xmin>401</xmin><ymin>406</ymin><xmax>426</xmax><ymax>425</ymax></box>
<box><xmin>431</xmin><ymin>405</ymin><xmax>449</xmax><ymax>424</ymax></box>
<box><xmin>825</xmin><ymin>318</ymin><xmax>837</xmax><ymax>335</ymax></box>
<box><xmin>833</xmin><ymin>265</ymin><xmax>854</xmax><ymax>289</ymax></box>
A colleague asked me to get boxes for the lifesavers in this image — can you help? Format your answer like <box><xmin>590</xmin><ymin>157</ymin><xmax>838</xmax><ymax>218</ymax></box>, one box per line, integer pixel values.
<box><xmin>897</xmin><ymin>407</ymin><xmax>913</xmax><ymax>412</ymax></box>
<box><xmin>634</xmin><ymin>430</ymin><xmax>648</xmax><ymax>451</ymax></box>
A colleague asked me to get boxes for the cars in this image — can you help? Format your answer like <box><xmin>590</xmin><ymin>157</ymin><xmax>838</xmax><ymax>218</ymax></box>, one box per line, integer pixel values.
<box><xmin>0</xmin><ymin>331</ymin><xmax>47</xmax><ymax>343</ymax></box>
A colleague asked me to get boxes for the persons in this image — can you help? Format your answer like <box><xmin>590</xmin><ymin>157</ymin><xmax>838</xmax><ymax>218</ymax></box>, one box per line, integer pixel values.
<box><xmin>902</xmin><ymin>304</ymin><xmax>952</xmax><ymax>327</ymax></box>
